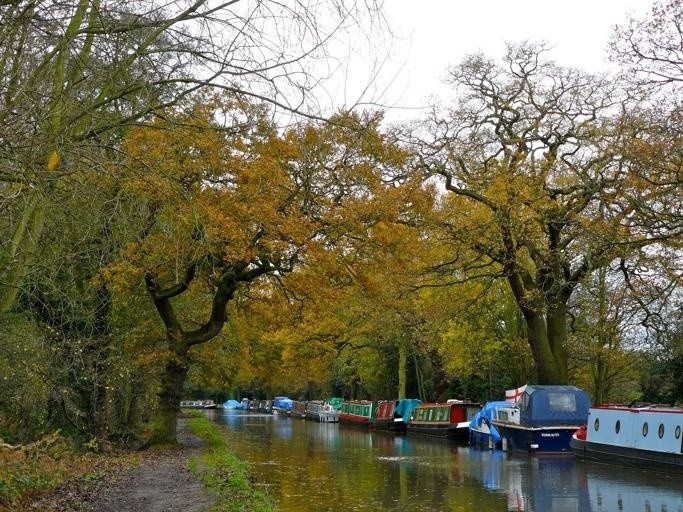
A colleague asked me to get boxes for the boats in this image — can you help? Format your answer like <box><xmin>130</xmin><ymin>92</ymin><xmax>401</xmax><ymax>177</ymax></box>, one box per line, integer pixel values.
<box><xmin>468</xmin><ymin>384</ymin><xmax>590</xmax><ymax>463</ymax></box>
<box><xmin>403</xmin><ymin>397</ymin><xmax>484</xmax><ymax>446</ymax></box>
<box><xmin>337</xmin><ymin>399</ymin><xmax>405</xmax><ymax>431</ymax></box>
<box><xmin>179</xmin><ymin>397</ymin><xmax>342</xmax><ymax>423</ymax></box>
<box><xmin>570</xmin><ymin>402</ymin><xmax>682</xmax><ymax>478</ymax></box>
<box><xmin>468</xmin><ymin>451</ymin><xmax>590</xmax><ymax>512</ymax></box>
<box><xmin>579</xmin><ymin>457</ymin><xmax>682</xmax><ymax>512</ymax></box>
